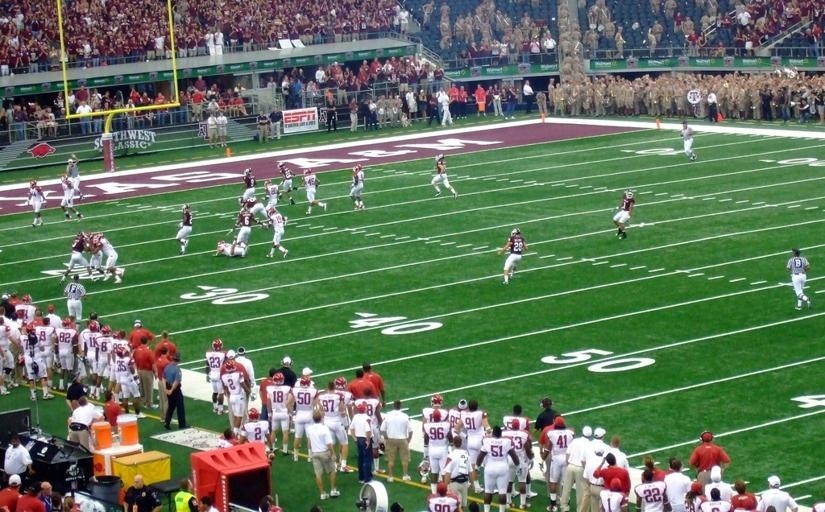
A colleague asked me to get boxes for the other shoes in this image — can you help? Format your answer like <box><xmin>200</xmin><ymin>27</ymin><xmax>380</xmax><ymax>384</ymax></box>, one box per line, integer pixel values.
<box><xmin>294</xmin><ymin>186</ymin><xmax>297</xmax><ymax>190</ymax></box>
<box><xmin>215</xmin><ymin>145</ymin><xmax>219</xmax><ymax>148</ymax></box>
<box><xmin>323</xmin><ymin>203</ymin><xmax>327</xmax><ymax>211</ymax></box>
<box><xmin>224</xmin><ymin>144</ymin><xmax>227</xmax><ymax>147</ymax></box>
<box><xmin>209</xmin><ymin>146</ymin><xmax>213</xmax><ymax>149</ymax></box>
<box><xmin>359</xmin><ymin>205</ymin><xmax>365</xmax><ymax>210</ymax></box>
<box><xmin>79</xmin><ymin>195</ymin><xmax>85</xmax><ymax>201</ymax></box>
<box><xmin>221</xmin><ymin>144</ymin><xmax>224</xmax><ymax>147</ymax></box>
<box><xmin>290</xmin><ymin>202</ymin><xmax>295</xmax><ymax>205</ymax></box>
<box><xmin>353</xmin><ymin>204</ymin><xmax>359</xmax><ymax>211</ymax></box>
<box><xmin>306</xmin><ymin>211</ymin><xmax>311</xmax><ymax>215</ymax></box>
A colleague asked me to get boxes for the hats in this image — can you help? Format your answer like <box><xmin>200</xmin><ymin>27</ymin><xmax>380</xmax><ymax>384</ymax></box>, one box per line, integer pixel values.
<box><xmin>552</xmin><ymin>416</ymin><xmax>565</xmax><ymax>427</ymax></box>
<box><xmin>226</xmin><ymin>349</ymin><xmax>237</xmax><ymax>359</ymax></box>
<box><xmin>432</xmin><ymin>409</ymin><xmax>442</xmax><ymax>421</ymax></box>
<box><xmin>6</xmin><ymin>473</ymin><xmax>23</xmax><ymax>486</ymax></box>
<box><xmin>680</xmin><ymin>120</ymin><xmax>688</xmax><ymax>125</ymax></box>
<box><xmin>700</xmin><ymin>431</ymin><xmax>714</xmax><ymax>442</ymax></box>
<box><xmin>133</xmin><ymin>320</ymin><xmax>141</xmax><ymax>326</ymax></box>
<box><xmin>581</xmin><ymin>425</ymin><xmax>606</xmax><ymax>437</ymax></box>
<box><xmin>66</xmin><ymin>159</ymin><xmax>73</xmax><ymax>163</ymax></box>
<box><xmin>539</xmin><ymin>397</ymin><xmax>554</xmax><ymax>406</ymax></box>
<box><xmin>436</xmin><ymin>480</ymin><xmax>449</xmax><ymax>495</ymax></box>
<box><xmin>70</xmin><ymin>274</ymin><xmax>79</xmax><ymax>279</ymax></box>
<box><xmin>710</xmin><ymin>464</ymin><xmax>722</xmax><ymax>482</ymax></box>
<box><xmin>791</xmin><ymin>247</ymin><xmax>802</xmax><ymax>256</ymax></box>
<box><xmin>512</xmin><ymin>418</ymin><xmax>520</xmax><ymax>429</ymax></box>
<box><xmin>766</xmin><ymin>475</ymin><xmax>783</xmax><ymax>488</ymax></box>
<box><xmin>691</xmin><ymin>481</ymin><xmax>704</xmax><ymax>492</ymax></box>
<box><xmin>282</xmin><ymin>356</ymin><xmax>291</xmax><ymax>364</ymax></box>
<box><xmin>357</xmin><ymin>401</ymin><xmax>367</xmax><ymax>413</ymax></box>
<box><xmin>609</xmin><ymin>478</ymin><xmax>622</xmax><ymax>491</ymax></box>
<box><xmin>302</xmin><ymin>366</ymin><xmax>314</xmax><ymax>376</ymax></box>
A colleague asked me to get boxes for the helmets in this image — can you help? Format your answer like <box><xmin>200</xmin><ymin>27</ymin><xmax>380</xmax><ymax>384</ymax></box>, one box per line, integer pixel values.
<box><xmin>20</xmin><ymin>292</ymin><xmax>129</xmax><ymax>358</ymax></box>
<box><xmin>182</xmin><ymin>204</ymin><xmax>190</xmax><ymax>213</ymax></box>
<box><xmin>510</xmin><ymin>228</ymin><xmax>522</xmax><ymax>237</ymax></box>
<box><xmin>270</xmin><ymin>372</ymin><xmax>286</xmax><ymax>385</ymax></box>
<box><xmin>624</xmin><ymin>189</ymin><xmax>634</xmax><ymax>199</ymax></box>
<box><xmin>333</xmin><ymin>376</ymin><xmax>349</xmax><ymax>390</ymax></box>
<box><xmin>299</xmin><ymin>376</ymin><xmax>313</xmax><ymax>388</ymax></box>
<box><xmin>429</xmin><ymin>393</ymin><xmax>445</xmax><ymax>406</ymax></box>
<box><xmin>353</xmin><ymin>166</ymin><xmax>359</xmax><ymax>173</ymax></box>
<box><xmin>61</xmin><ymin>175</ymin><xmax>67</xmax><ymax>182</ymax></box>
<box><xmin>210</xmin><ymin>337</ymin><xmax>223</xmax><ymax>351</ymax></box>
<box><xmin>277</xmin><ymin>162</ymin><xmax>285</xmax><ymax>169</ymax></box>
<box><xmin>356</xmin><ymin>164</ymin><xmax>362</xmax><ymax>169</ymax></box>
<box><xmin>239</xmin><ymin>168</ymin><xmax>276</xmax><ymax>215</ymax></box>
<box><xmin>247</xmin><ymin>408</ymin><xmax>259</xmax><ymax>422</ymax></box>
<box><xmin>434</xmin><ymin>153</ymin><xmax>445</xmax><ymax>161</ymax></box>
<box><xmin>30</xmin><ymin>180</ymin><xmax>36</xmax><ymax>187</ymax></box>
<box><xmin>304</xmin><ymin>168</ymin><xmax>311</xmax><ymax>174</ymax></box>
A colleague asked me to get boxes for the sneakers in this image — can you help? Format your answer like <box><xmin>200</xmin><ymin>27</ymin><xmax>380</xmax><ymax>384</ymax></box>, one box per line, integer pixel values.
<box><xmin>467</xmin><ymin>480</ymin><xmax>571</xmax><ymax>512</ymax></box>
<box><xmin>319</xmin><ymin>493</ymin><xmax>329</xmax><ymax>499</ymax></box>
<box><xmin>615</xmin><ymin>228</ymin><xmax>621</xmax><ymax>236</ymax></box>
<box><xmin>807</xmin><ymin>296</ymin><xmax>813</xmax><ymax>311</ymax></box>
<box><xmin>179</xmin><ymin>239</ymin><xmax>293</xmax><ymax>261</ymax></box>
<box><xmin>500</xmin><ymin>281</ymin><xmax>509</xmax><ymax>285</ymax></box>
<box><xmin>618</xmin><ymin>232</ymin><xmax>627</xmax><ymax>240</ymax></box>
<box><xmin>330</xmin><ymin>490</ymin><xmax>341</xmax><ymax>497</ymax></box>
<box><xmin>453</xmin><ymin>192</ymin><xmax>459</xmax><ymax>199</ymax></box>
<box><xmin>510</xmin><ymin>275</ymin><xmax>514</xmax><ymax>279</ymax></box>
<box><xmin>434</xmin><ymin>190</ymin><xmax>442</xmax><ymax>197</ymax></box>
<box><xmin>0</xmin><ymin>378</ymin><xmax>192</xmax><ymax>431</ymax></box>
<box><xmin>270</xmin><ymin>447</ymin><xmax>312</xmax><ymax>463</ymax></box>
<box><xmin>60</xmin><ymin>267</ymin><xmax>125</xmax><ymax>284</ymax></box>
<box><xmin>688</xmin><ymin>153</ymin><xmax>697</xmax><ymax>162</ymax></box>
<box><xmin>794</xmin><ymin>306</ymin><xmax>803</xmax><ymax>311</ymax></box>
<box><xmin>212</xmin><ymin>406</ymin><xmax>229</xmax><ymax>415</ymax></box>
<box><xmin>336</xmin><ymin>460</ymin><xmax>431</xmax><ymax>486</ymax></box>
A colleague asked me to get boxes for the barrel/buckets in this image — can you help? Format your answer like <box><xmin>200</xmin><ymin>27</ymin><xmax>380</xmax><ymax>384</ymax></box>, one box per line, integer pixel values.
<box><xmin>87</xmin><ymin>474</ymin><xmax>124</xmax><ymax>504</ymax></box>
<box><xmin>93</xmin><ymin>422</ymin><xmax>112</xmax><ymax>450</ymax></box>
<box><xmin>116</xmin><ymin>414</ymin><xmax>138</xmax><ymax>446</ymax></box>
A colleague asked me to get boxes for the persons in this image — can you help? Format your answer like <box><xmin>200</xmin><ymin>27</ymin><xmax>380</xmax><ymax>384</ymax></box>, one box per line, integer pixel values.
<box><xmin>173</xmin><ymin>203</ymin><xmax>193</xmax><ymax>256</ymax></box>
<box><xmin>63</xmin><ymin>274</ymin><xmax>87</xmax><ymax>332</ymax></box>
<box><xmin>1</xmin><ymin>0</ymin><xmax>824</xmax><ymax>134</ymax></box>
<box><xmin>58</xmin><ymin>173</ymin><xmax>84</xmax><ymax>223</ymax></box>
<box><xmin>24</xmin><ymin>181</ymin><xmax>48</xmax><ymax>228</ymax></box>
<box><xmin>785</xmin><ymin>248</ymin><xmax>812</xmax><ymax>313</ymax></box>
<box><xmin>349</xmin><ymin>163</ymin><xmax>364</xmax><ymax>211</ymax></box>
<box><xmin>296</xmin><ymin>169</ymin><xmax>327</xmax><ymax>216</ymax></box>
<box><xmin>497</xmin><ymin>228</ymin><xmax>529</xmax><ymax>286</ymax></box>
<box><xmin>211</xmin><ymin>162</ymin><xmax>296</xmax><ymax>260</ymax></box>
<box><xmin>356</xmin><ymin>164</ymin><xmax>364</xmax><ymax>183</ymax></box>
<box><xmin>429</xmin><ymin>153</ymin><xmax>458</xmax><ymax>202</ymax></box>
<box><xmin>680</xmin><ymin>120</ymin><xmax>698</xmax><ymax>163</ymax></box>
<box><xmin>89</xmin><ymin>233</ymin><xmax>126</xmax><ymax>278</ymax></box>
<box><xmin>59</xmin><ymin>233</ymin><xmax>100</xmax><ymax>282</ymax></box>
<box><xmin>612</xmin><ymin>189</ymin><xmax>636</xmax><ymax>241</ymax></box>
<box><xmin>85</xmin><ymin>233</ymin><xmax>123</xmax><ymax>284</ymax></box>
<box><xmin>64</xmin><ymin>154</ymin><xmax>85</xmax><ymax>202</ymax></box>
